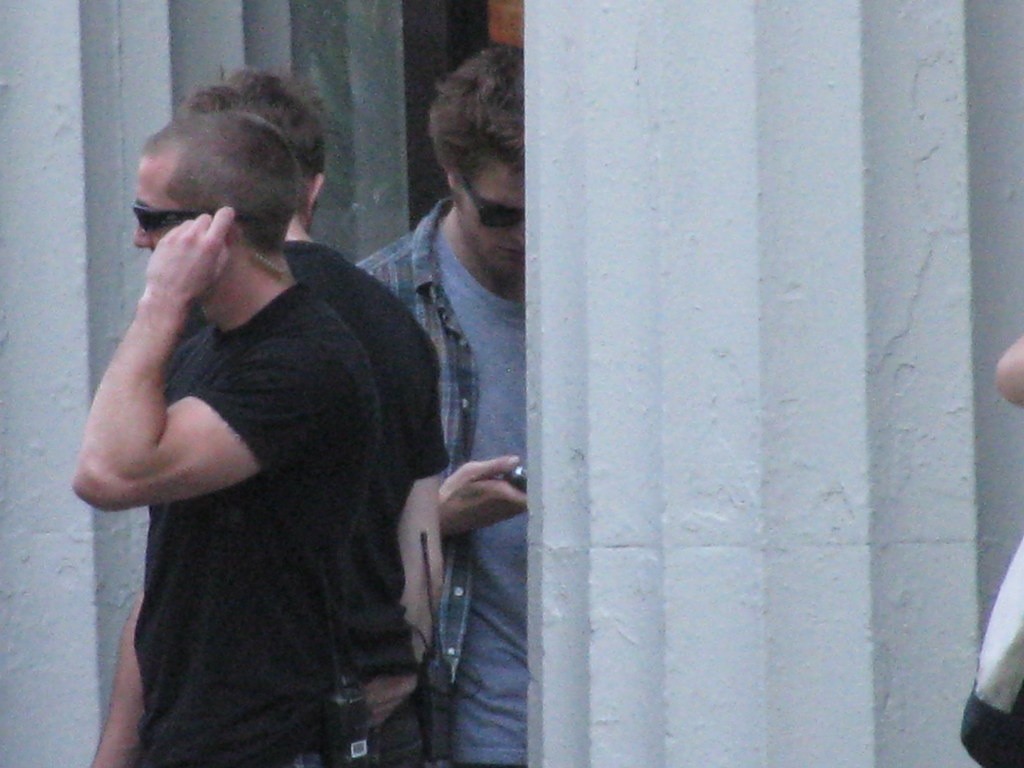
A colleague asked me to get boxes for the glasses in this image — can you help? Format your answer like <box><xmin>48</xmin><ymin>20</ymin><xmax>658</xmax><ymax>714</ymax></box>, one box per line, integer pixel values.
<box><xmin>132</xmin><ymin>204</ymin><xmax>248</xmax><ymax>234</ymax></box>
<box><xmin>463</xmin><ymin>175</ymin><xmax>524</xmax><ymax>227</ymax></box>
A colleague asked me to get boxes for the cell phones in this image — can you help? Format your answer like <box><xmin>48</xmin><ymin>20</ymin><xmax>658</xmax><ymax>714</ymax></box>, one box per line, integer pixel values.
<box><xmin>503</xmin><ymin>466</ymin><xmax>528</xmax><ymax>494</ymax></box>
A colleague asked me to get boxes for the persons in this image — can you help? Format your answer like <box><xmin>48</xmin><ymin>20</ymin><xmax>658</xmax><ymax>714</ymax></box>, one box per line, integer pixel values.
<box><xmin>354</xmin><ymin>39</ymin><xmax>528</xmax><ymax>768</ymax></box>
<box><xmin>73</xmin><ymin>115</ymin><xmax>378</xmax><ymax>768</ymax></box>
<box><xmin>962</xmin><ymin>330</ymin><xmax>1023</xmax><ymax>768</ymax></box>
<box><xmin>179</xmin><ymin>66</ymin><xmax>448</xmax><ymax>768</ymax></box>
